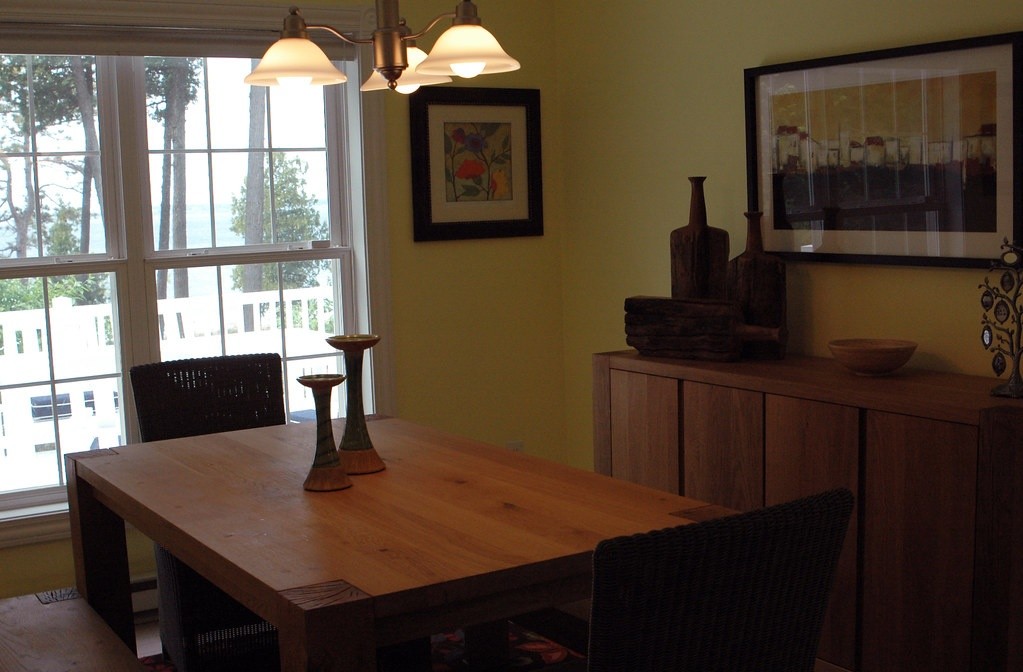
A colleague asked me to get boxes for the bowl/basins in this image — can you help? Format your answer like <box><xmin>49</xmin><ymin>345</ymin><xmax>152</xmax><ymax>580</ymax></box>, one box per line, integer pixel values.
<box><xmin>828</xmin><ymin>338</ymin><xmax>918</xmax><ymax>377</ymax></box>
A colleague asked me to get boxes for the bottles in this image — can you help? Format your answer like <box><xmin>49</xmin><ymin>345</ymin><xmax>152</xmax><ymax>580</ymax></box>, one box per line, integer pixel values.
<box><xmin>729</xmin><ymin>212</ymin><xmax>790</xmax><ymax>331</ymax></box>
<box><xmin>669</xmin><ymin>175</ymin><xmax>730</xmax><ymax>301</ymax></box>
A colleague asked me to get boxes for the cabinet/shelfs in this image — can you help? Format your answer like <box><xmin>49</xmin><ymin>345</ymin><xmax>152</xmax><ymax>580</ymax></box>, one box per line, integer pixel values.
<box><xmin>590</xmin><ymin>352</ymin><xmax>1023</xmax><ymax>672</ymax></box>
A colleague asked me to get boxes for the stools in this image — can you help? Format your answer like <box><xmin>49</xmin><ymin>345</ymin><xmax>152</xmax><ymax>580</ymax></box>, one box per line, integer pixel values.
<box><xmin>0</xmin><ymin>588</ymin><xmax>152</xmax><ymax>672</ymax></box>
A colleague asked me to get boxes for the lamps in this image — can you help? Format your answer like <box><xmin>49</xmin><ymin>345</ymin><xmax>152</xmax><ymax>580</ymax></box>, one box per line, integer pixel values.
<box><xmin>244</xmin><ymin>0</ymin><xmax>521</xmax><ymax>96</ymax></box>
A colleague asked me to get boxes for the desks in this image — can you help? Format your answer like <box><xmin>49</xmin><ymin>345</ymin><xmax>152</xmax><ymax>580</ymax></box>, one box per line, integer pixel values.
<box><xmin>63</xmin><ymin>410</ymin><xmax>747</xmax><ymax>672</ymax></box>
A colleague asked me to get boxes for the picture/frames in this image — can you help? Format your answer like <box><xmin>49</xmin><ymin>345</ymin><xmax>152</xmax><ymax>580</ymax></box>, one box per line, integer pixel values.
<box><xmin>743</xmin><ymin>29</ymin><xmax>1023</xmax><ymax>270</ymax></box>
<box><xmin>405</xmin><ymin>86</ymin><xmax>545</xmax><ymax>244</ymax></box>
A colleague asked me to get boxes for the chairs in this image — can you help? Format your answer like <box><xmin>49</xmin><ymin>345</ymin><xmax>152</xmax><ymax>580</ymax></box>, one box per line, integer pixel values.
<box><xmin>126</xmin><ymin>352</ymin><xmax>281</xmax><ymax>671</ymax></box>
<box><xmin>454</xmin><ymin>485</ymin><xmax>856</xmax><ymax>672</ymax></box>
